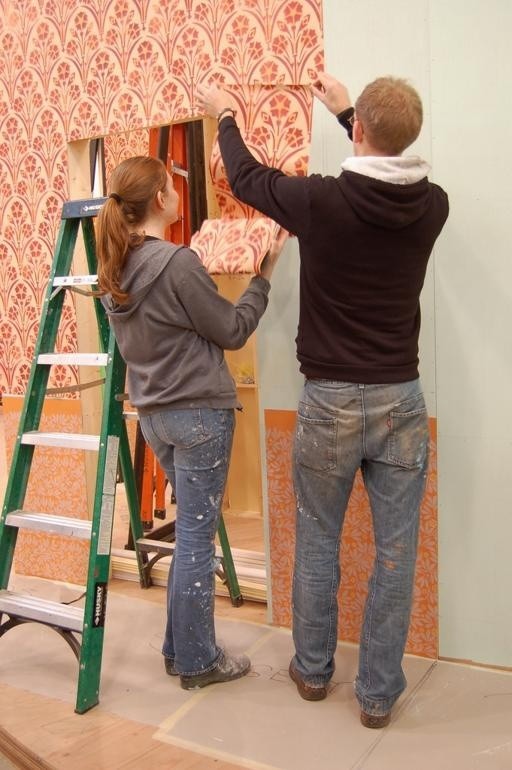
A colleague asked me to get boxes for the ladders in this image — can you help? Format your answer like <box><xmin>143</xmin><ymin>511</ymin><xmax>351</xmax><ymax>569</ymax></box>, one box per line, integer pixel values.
<box><xmin>3</xmin><ymin>197</ymin><xmax>243</xmax><ymax>716</ymax></box>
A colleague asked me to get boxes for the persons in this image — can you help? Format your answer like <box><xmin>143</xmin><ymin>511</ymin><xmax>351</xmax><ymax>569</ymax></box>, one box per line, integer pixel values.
<box><xmin>96</xmin><ymin>156</ymin><xmax>289</xmax><ymax>692</ymax></box>
<box><xmin>192</xmin><ymin>73</ymin><xmax>450</xmax><ymax>728</ymax></box>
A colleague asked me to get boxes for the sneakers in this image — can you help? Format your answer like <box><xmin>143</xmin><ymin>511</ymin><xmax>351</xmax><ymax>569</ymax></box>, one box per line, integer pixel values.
<box><xmin>289</xmin><ymin>655</ymin><xmax>327</xmax><ymax>701</ymax></box>
<box><xmin>360</xmin><ymin>709</ymin><xmax>390</xmax><ymax>727</ymax></box>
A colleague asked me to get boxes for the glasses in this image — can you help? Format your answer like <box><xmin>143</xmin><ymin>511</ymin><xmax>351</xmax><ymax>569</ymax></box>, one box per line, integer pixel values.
<box><xmin>348</xmin><ymin>116</ymin><xmax>364</xmax><ymax>134</ymax></box>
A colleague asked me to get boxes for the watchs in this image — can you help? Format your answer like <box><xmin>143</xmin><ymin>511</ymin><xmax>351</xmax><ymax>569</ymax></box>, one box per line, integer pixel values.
<box><xmin>217</xmin><ymin>108</ymin><xmax>237</xmax><ymax>122</ymax></box>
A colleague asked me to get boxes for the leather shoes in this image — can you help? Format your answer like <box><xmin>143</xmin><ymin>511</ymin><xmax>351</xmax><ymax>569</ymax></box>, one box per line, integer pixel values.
<box><xmin>160</xmin><ymin>638</ymin><xmax>225</xmax><ymax>677</ymax></box>
<box><xmin>181</xmin><ymin>654</ymin><xmax>251</xmax><ymax>690</ymax></box>
